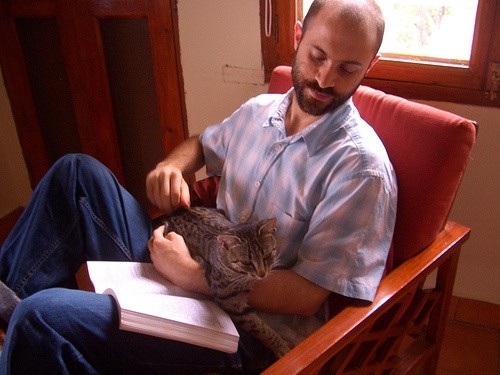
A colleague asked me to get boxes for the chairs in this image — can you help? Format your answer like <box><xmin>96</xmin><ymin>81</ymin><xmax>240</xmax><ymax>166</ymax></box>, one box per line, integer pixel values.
<box><xmin>145</xmin><ymin>64</ymin><xmax>479</xmax><ymax>375</ymax></box>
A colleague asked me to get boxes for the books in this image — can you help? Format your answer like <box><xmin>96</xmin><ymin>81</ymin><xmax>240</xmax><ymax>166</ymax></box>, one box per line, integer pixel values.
<box><xmin>86</xmin><ymin>260</ymin><xmax>240</xmax><ymax>355</ymax></box>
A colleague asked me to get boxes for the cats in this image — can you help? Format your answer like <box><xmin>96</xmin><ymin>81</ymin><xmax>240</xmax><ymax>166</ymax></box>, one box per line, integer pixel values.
<box><xmin>151</xmin><ymin>207</ymin><xmax>292</xmax><ymax>359</ymax></box>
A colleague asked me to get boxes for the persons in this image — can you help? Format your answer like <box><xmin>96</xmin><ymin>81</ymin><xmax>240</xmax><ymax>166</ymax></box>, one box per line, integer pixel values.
<box><xmin>0</xmin><ymin>0</ymin><xmax>398</xmax><ymax>375</ymax></box>
